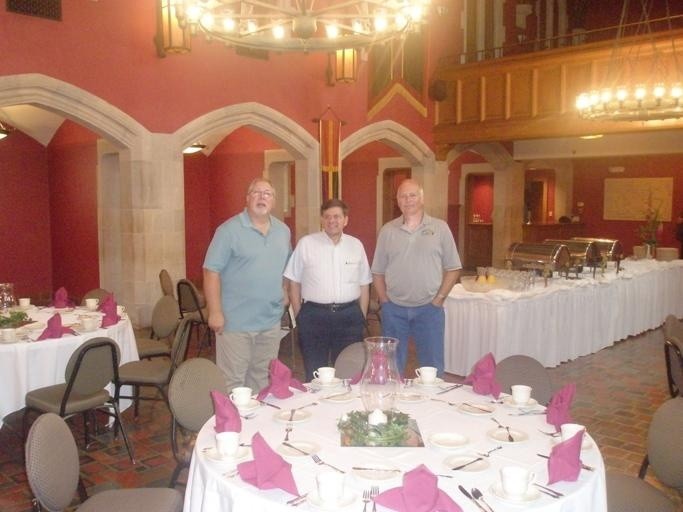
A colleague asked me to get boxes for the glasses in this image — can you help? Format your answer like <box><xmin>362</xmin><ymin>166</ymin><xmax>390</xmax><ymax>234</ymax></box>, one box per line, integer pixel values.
<box><xmin>247</xmin><ymin>189</ymin><xmax>274</xmax><ymax>200</ymax></box>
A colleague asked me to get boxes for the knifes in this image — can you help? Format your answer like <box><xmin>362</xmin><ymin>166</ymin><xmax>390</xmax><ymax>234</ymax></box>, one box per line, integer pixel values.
<box><xmin>353</xmin><ymin>466</ymin><xmax>403</xmax><ymax>473</ymax></box>
<box><xmin>438</xmin><ymin>384</ymin><xmax>463</xmax><ymax>396</ymax></box>
<box><xmin>283</xmin><ymin>441</ymin><xmax>311</xmax><ymax>455</ymax></box>
<box><xmin>458</xmin><ymin>484</ymin><xmax>487</xmax><ymax>512</ymax></box>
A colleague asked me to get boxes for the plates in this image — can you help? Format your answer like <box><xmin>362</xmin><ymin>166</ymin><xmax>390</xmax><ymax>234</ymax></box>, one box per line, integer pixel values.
<box><xmin>233</xmin><ymin>399</ymin><xmax>261</xmax><ymax>409</ymax></box>
<box><xmin>488</xmin><ymin>428</ymin><xmax>530</xmax><ymax>445</ymax></box>
<box><xmin>428</xmin><ymin>431</ymin><xmax>470</xmax><ymax>449</ymax></box>
<box><xmin>500</xmin><ymin>396</ymin><xmax>538</xmax><ymax>407</ymax></box>
<box><xmin>444</xmin><ymin>453</ymin><xmax>495</xmax><ymax>474</ymax></box>
<box><xmin>414</xmin><ymin>377</ymin><xmax>443</xmax><ymax>387</ymax></box>
<box><xmin>280</xmin><ymin>444</ymin><xmax>313</xmax><ymax>458</ymax></box>
<box><xmin>209</xmin><ymin>445</ymin><xmax>250</xmax><ymax>462</ymax></box>
<box><xmin>359</xmin><ymin>463</ymin><xmax>399</xmax><ymax>481</ymax></box>
<box><xmin>312</xmin><ymin>378</ymin><xmax>341</xmax><ymax>389</ymax></box>
<box><xmin>456</xmin><ymin>401</ymin><xmax>496</xmax><ymax>416</ymax></box>
<box><xmin>307</xmin><ymin>486</ymin><xmax>356</xmax><ymax>510</ymax></box>
<box><xmin>489</xmin><ymin>480</ymin><xmax>541</xmax><ymax>504</ymax></box>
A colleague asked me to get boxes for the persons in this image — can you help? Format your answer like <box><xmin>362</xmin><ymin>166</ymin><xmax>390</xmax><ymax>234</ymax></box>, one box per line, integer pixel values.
<box><xmin>203</xmin><ymin>178</ymin><xmax>293</xmax><ymax>396</ymax></box>
<box><xmin>282</xmin><ymin>199</ymin><xmax>372</xmax><ymax>383</ymax></box>
<box><xmin>371</xmin><ymin>178</ymin><xmax>462</xmax><ymax>380</ymax></box>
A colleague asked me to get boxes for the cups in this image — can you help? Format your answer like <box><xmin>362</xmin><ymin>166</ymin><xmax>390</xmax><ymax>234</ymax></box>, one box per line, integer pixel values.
<box><xmin>510</xmin><ymin>384</ymin><xmax>532</xmax><ymax>404</ymax></box>
<box><xmin>315</xmin><ymin>470</ymin><xmax>346</xmax><ymax>501</ymax></box>
<box><xmin>415</xmin><ymin>366</ymin><xmax>438</xmax><ymax>383</ymax></box>
<box><xmin>230</xmin><ymin>386</ymin><xmax>253</xmax><ymax>405</ymax></box>
<box><xmin>216</xmin><ymin>432</ymin><xmax>239</xmax><ymax>456</ymax></box>
<box><xmin>573</xmin><ymin>265</ymin><xmax>583</xmax><ymax>272</ymax></box>
<box><xmin>313</xmin><ymin>367</ymin><xmax>336</xmax><ymax>384</ymax></box>
<box><xmin>0</xmin><ymin>298</ymin><xmax>126</xmax><ymax>339</ymax></box>
<box><xmin>458</xmin><ymin>264</ymin><xmax>537</xmax><ymax>294</ymax></box>
<box><xmin>500</xmin><ymin>467</ymin><xmax>536</xmax><ymax>496</ymax></box>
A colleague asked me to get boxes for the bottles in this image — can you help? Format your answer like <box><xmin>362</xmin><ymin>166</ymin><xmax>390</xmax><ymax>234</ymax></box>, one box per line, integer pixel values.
<box><xmin>361</xmin><ymin>336</ymin><xmax>403</xmax><ymax>413</ymax></box>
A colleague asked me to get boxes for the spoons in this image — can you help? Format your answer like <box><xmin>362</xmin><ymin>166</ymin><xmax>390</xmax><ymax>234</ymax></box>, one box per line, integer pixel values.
<box><xmin>439</xmin><ymin>384</ymin><xmax>458</xmax><ymax>390</ymax></box>
<box><xmin>491</xmin><ymin>418</ymin><xmax>504</xmax><ymax>428</ymax></box>
<box><xmin>507</xmin><ymin>427</ymin><xmax>514</xmax><ymax>442</ymax></box>
<box><xmin>471</xmin><ymin>487</ymin><xmax>494</xmax><ymax>512</ymax></box>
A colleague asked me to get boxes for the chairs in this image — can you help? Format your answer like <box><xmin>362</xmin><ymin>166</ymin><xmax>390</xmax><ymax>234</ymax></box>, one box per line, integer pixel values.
<box><xmin>26</xmin><ymin>335</ymin><xmax>134</xmax><ymax>466</ymax></box>
<box><xmin>136</xmin><ymin>296</ymin><xmax>181</xmax><ymax>361</ymax></box>
<box><xmin>111</xmin><ymin>315</ymin><xmax>196</xmax><ymax>436</ymax></box>
<box><xmin>159</xmin><ymin>270</ymin><xmax>205</xmax><ymax>318</ymax></box>
<box><xmin>182</xmin><ymin>378</ymin><xmax>609</xmax><ymax>512</ymax></box>
<box><xmin>494</xmin><ymin>355</ymin><xmax>552</xmax><ymax>409</ymax></box>
<box><xmin>174</xmin><ymin>277</ymin><xmax>214</xmax><ymax>351</ymax></box>
<box><xmin>335</xmin><ymin>341</ymin><xmax>396</xmax><ymax>381</ymax></box>
<box><xmin>662</xmin><ymin>314</ymin><xmax>683</xmax><ymax>398</ymax></box>
<box><xmin>605</xmin><ymin>394</ymin><xmax>683</xmax><ymax>512</ymax></box>
<box><xmin>167</xmin><ymin>356</ymin><xmax>228</xmax><ymax>485</ymax></box>
<box><xmin>23</xmin><ymin>412</ymin><xmax>184</xmax><ymax>510</ymax></box>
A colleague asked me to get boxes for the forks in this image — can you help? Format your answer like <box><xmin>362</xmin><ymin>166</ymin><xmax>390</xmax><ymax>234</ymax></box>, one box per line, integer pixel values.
<box><xmin>362</xmin><ymin>490</ymin><xmax>370</xmax><ymax>512</ymax></box>
<box><xmin>313</xmin><ymin>455</ymin><xmax>346</xmax><ymax>474</ymax></box>
<box><xmin>370</xmin><ymin>486</ymin><xmax>380</xmax><ymax>512</ymax></box>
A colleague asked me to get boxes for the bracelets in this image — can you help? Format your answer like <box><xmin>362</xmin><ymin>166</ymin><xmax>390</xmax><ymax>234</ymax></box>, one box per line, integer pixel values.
<box><xmin>438</xmin><ymin>293</ymin><xmax>447</xmax><ymax>299</ymax></box>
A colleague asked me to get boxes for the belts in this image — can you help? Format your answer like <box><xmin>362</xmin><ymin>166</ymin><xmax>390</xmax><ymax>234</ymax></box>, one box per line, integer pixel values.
<box><xmin>306</xmin><ymin>300</ymin><xmax>356</xmax><ymax>313</ymax></box>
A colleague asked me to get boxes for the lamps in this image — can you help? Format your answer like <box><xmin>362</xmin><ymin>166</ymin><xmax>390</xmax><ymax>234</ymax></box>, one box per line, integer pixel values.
<box><xmin>334</xmin><ymin>48</ymin><xmax>358</xmax><ymax>84</ymax></box>
<box><xmin>175</xmin><ymin>0</ymin><xmax>434</xmax><ymax>55</ymax></box>
<box><xmin>0</xmin><ymin>119</ymin><xmax>12</xmax><ymax>142</ymax></box>
<box><xmin>574</xmin><ymin>0</ymin><xmax>683</xmax><ymax>123</ymax></box>
<box><xmin>156</xmin><ymin>0</ymin><xmax>189</xmax><ymax>53</ymax></box>
<box><xmin>183</xmin><ymin>136</ymin><xmax>206</xmax><ymax>154</ymax></box>
